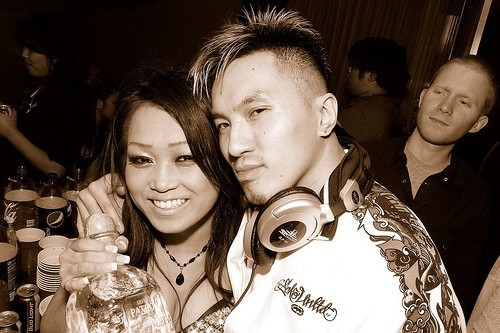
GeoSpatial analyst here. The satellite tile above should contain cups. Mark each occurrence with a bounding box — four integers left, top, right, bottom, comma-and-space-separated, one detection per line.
36, 248, 65, 321
38, 235, 70, 252
16, 227, 46, 272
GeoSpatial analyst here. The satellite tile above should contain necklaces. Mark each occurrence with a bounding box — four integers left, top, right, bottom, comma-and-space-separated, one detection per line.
158, 234, 210, 286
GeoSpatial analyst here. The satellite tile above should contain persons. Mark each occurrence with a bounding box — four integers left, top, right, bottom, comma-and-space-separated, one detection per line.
80, 79, 121, 175
75, 6, 466, 333
335, 34, 413, 146
0, 10, 91, 182
37, 59, 252, 333
365, 51, 500, 321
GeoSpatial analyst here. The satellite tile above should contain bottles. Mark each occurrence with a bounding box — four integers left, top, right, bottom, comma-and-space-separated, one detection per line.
65, 213, 176, 333
35, 172, 77, 239
0, 216, 19, 313
2, 164, 39, 230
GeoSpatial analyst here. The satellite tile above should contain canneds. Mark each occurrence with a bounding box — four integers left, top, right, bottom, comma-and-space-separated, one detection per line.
0, 311, 22, 333
0, 105, 12, 116
14, 284, 41, 333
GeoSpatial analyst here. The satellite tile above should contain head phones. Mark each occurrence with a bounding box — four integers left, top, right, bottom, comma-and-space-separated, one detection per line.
241, 132, 375, 270
